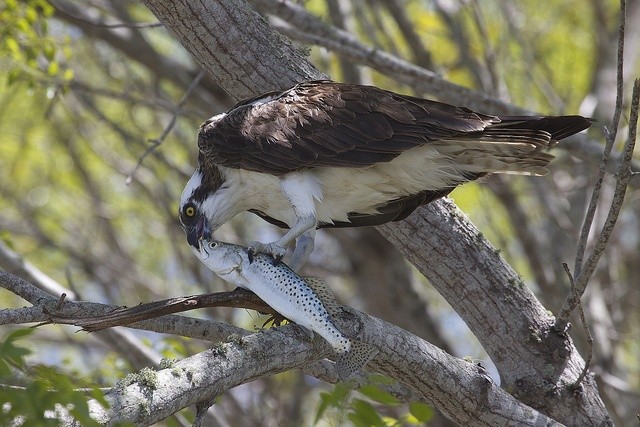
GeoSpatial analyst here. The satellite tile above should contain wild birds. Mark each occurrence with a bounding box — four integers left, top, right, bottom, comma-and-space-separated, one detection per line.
177, 79, 597, 268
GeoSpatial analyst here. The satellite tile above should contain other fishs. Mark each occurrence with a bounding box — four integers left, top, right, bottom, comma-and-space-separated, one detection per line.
188, 238, 380, 383
